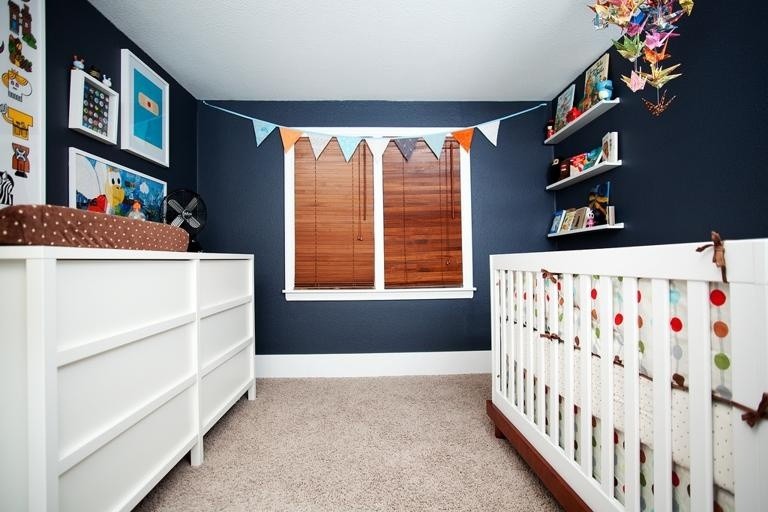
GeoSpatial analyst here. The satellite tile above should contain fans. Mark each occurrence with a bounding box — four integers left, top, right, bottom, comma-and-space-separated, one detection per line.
158, 189, 206, 240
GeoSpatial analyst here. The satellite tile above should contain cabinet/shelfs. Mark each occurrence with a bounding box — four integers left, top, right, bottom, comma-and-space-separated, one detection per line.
1, 247, 254, 511
544, 91, 626, 238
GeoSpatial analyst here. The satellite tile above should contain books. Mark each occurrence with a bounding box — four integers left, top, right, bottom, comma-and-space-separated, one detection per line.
570, 132, 618, 178
583, 53, 610, 98
549, 181, 616, 234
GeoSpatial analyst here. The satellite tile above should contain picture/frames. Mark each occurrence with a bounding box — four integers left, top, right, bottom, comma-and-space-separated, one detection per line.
70, 69, 120, 145
120, 46, 172, 169
67, 147, 168, 221
